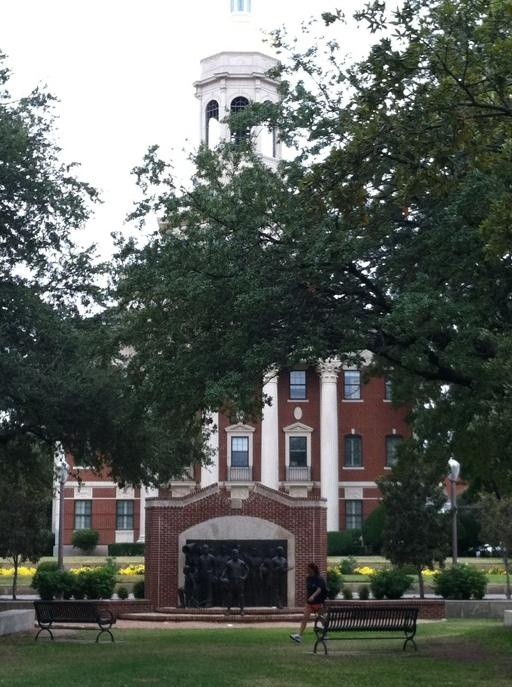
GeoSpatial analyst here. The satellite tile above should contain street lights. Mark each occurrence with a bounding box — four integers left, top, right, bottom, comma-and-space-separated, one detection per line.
447, 458, 463, 562
55, 457, 68, 568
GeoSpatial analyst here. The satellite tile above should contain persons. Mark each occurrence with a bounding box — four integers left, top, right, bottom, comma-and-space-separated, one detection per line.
219, 548, 250, 615
175, 565, 200, 608
269, 545, 294, 609
289, 561, 328, 644
197, 543, 218, 608
182, 541, 275, 606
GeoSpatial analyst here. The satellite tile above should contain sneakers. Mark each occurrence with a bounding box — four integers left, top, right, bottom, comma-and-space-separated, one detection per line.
319, 633, 327, 640
289, 634, 302, 644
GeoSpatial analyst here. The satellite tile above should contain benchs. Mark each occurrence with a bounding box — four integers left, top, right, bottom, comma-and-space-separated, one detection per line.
33, 600, 117, 644
313, 606, 419, 654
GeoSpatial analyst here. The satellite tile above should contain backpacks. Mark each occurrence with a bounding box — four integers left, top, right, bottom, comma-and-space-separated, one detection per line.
320, 576, 328, 601
